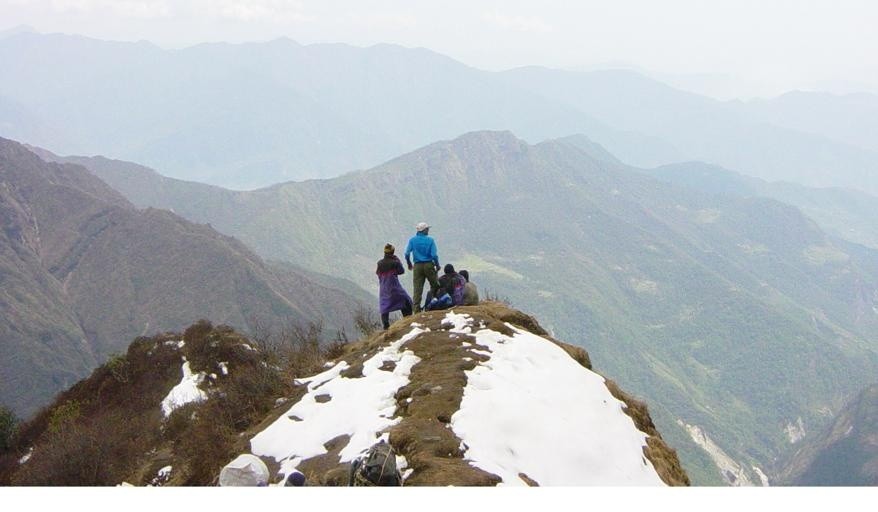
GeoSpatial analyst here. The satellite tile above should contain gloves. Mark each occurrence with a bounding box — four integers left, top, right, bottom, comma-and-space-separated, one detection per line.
408, 264, 413, 271
435, 262, 441, 272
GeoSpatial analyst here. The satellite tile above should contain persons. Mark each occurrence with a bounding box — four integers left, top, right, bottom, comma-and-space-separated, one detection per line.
376, 243, 412, 330
405, 222, 478, 314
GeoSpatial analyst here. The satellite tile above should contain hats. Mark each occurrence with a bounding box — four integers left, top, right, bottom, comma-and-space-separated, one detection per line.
384, 243, 395, 254
444, 264, 454, 274
459, 270, 469, 282
416, 223, 432, 231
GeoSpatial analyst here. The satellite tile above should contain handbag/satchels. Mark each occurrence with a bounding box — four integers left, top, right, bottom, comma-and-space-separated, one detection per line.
422, 288, 454, 311
